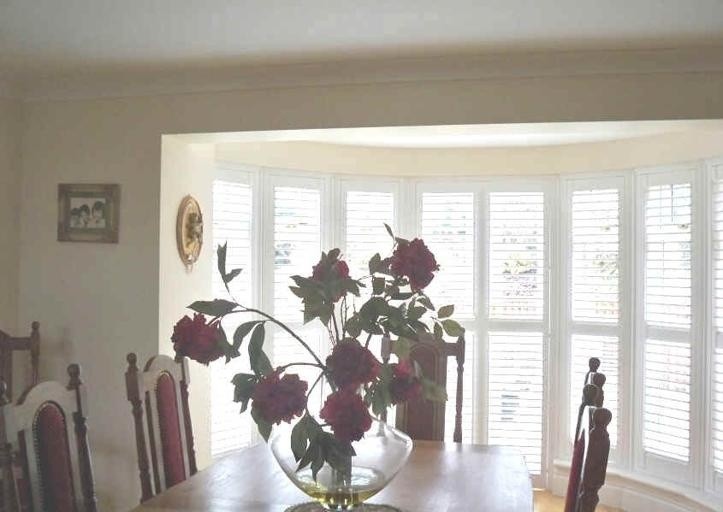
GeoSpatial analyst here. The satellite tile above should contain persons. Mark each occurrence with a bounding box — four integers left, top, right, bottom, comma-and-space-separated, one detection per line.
83, 200, 106, 229
73, 204, 90, 228
70, 207, 80, 227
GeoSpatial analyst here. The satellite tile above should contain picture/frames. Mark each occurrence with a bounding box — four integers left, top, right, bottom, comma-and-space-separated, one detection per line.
57, 182, 121, 245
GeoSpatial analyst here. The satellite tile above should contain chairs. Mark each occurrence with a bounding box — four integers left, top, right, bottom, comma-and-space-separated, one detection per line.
375, 325, 469, 444
563, 355, 613, 512
0, 320, 198, 511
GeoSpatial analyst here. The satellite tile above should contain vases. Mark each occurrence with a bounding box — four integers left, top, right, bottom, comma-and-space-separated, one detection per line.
270, 409, 415, 510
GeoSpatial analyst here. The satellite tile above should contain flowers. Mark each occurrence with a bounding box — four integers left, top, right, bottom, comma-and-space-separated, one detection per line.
170, 220, 466, 489
